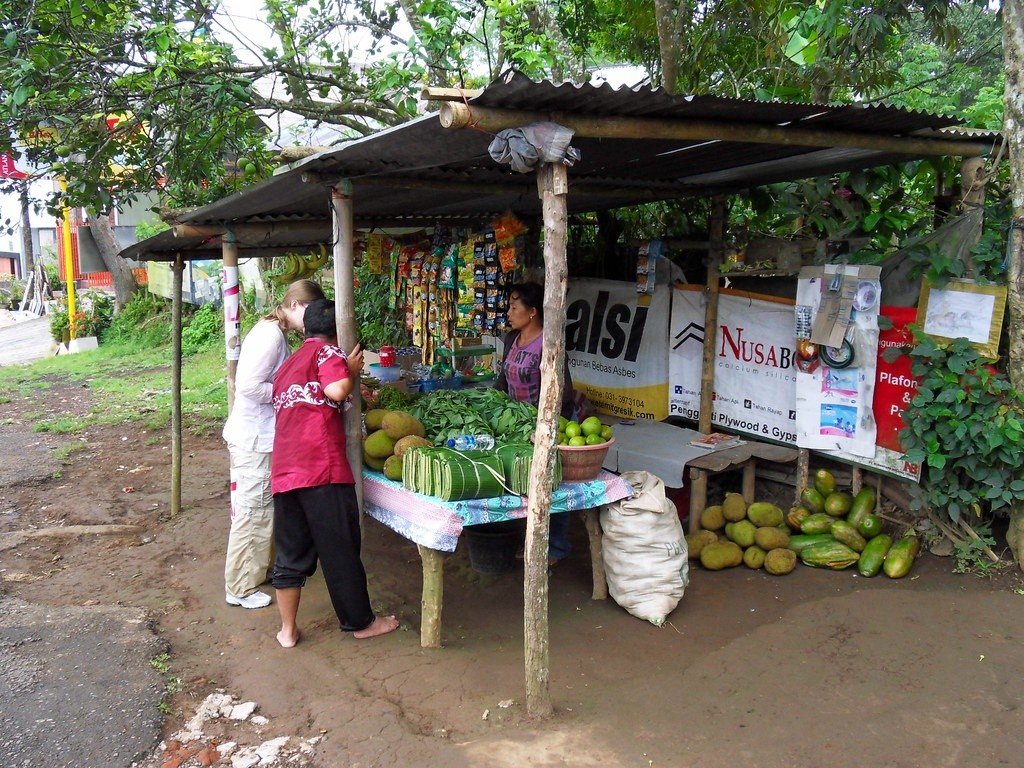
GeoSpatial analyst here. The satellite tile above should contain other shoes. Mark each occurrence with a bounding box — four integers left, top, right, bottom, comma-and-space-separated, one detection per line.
547, 551, 570, 570
513, 547, 524, 561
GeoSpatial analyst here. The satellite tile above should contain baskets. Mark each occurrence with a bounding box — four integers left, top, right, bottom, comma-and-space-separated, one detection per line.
417, 375, 464, 395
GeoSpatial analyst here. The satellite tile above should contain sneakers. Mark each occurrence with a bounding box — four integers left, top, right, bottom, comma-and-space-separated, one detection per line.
225, 591, 273, 610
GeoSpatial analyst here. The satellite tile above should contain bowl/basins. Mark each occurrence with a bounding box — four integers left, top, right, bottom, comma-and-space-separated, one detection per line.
368, 362, 400, 381
407, 383, 420, 394
433, 377, 449, 392
449, 375, 462, 392
419, 379, 435, 394
851, 280, 878, 313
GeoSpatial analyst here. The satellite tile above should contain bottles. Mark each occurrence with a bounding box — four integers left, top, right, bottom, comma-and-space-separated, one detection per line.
447, 434, 495, 451
393, 346, 420, 356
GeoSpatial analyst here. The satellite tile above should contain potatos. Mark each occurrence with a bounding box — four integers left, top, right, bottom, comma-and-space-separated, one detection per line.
364, 409, 432, 480
685, 494, 796, 573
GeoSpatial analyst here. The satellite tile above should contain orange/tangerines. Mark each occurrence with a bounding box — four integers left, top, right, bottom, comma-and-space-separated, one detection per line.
557, 416, 612, 445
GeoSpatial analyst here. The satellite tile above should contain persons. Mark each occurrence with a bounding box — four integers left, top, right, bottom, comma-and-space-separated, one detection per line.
493, 283, 573, 422
222, 280, 399, 648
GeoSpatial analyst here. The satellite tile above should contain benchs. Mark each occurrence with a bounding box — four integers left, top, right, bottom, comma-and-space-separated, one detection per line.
599, 416, 799, 535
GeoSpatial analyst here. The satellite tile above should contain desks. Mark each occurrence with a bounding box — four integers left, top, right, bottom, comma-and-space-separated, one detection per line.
362, 466, 639, 647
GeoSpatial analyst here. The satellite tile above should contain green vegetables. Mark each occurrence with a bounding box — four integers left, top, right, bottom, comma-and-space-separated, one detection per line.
376, 385, 538, 450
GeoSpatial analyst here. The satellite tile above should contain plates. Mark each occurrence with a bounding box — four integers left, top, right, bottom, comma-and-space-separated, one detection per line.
819, 337, 855, 369
